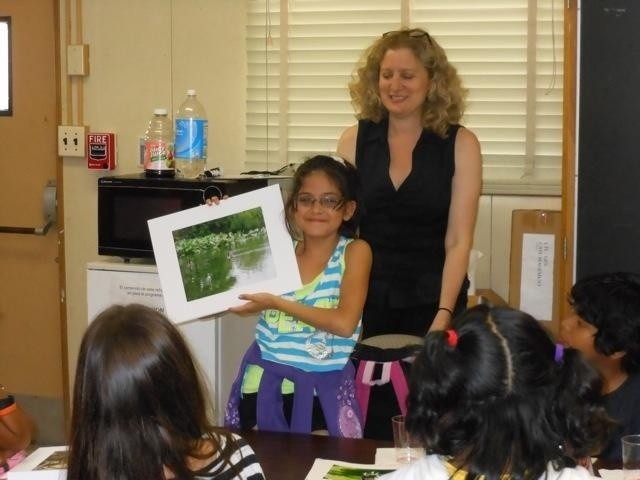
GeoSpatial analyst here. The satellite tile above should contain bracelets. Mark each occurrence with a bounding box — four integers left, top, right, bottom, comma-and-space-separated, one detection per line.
438, 307, 453, 315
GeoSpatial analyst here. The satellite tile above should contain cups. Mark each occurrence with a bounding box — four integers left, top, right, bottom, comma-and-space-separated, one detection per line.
391, 414, 425, 466
619, 433, 640, 480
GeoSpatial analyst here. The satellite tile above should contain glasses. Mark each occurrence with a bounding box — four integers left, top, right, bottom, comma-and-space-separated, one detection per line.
294, 194, 339, 208
383, 30, 432, 45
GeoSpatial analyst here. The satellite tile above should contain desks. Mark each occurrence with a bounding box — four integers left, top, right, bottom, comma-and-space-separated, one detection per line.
214, 425, 640, 480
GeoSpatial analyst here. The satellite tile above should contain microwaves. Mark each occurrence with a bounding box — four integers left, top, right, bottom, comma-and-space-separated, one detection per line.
95, 167, 297, 264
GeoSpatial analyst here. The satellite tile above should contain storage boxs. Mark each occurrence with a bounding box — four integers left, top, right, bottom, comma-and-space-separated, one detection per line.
510, 208, 564, 342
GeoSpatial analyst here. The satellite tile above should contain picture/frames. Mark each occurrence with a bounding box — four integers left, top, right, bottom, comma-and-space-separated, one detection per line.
146, 182, 303, 330
305, 457, 403, 480
6, 446, 72, 480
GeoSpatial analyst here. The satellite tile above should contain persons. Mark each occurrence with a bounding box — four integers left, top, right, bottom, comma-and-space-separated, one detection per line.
374, 302, 624, 480
206, 154, 374, 436
65, 302, 268, 480
335, 27, 485, 337
558, 270, 640, 470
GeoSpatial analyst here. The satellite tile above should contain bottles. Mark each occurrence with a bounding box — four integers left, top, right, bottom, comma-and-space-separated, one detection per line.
143, 107, 174, 177
173, 88, 209, 179
